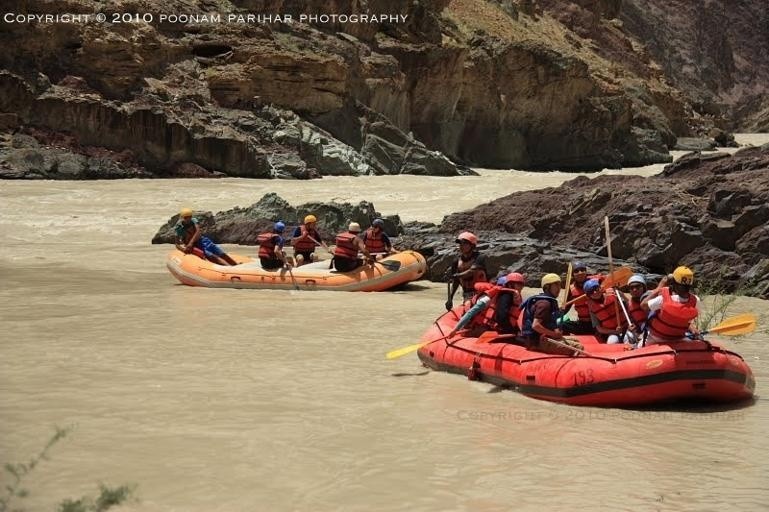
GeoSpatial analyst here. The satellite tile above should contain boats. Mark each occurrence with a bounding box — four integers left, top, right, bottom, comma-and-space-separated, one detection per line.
413, 292, 757, 413
164, 243, 430, 293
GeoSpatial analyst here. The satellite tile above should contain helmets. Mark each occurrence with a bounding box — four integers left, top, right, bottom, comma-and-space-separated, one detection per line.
672, 265, 694, 286
372, 219, 384, 225
274, 221, 286, 231
457, 232, 477, 245
304, 215, 316, 225
179, 208, 193, 218
541, 272, 562, 288
583, 278, 600, 294
572, 261, 586, 272
506, 272, 525, 286
348, 222, 361, 232
627, 275, 648, 290
497, 276, 508, 285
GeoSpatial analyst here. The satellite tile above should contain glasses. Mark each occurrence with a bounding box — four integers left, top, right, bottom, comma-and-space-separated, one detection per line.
574, 268, 584, 273
629, 283, 642, 290
588, 287, 599, 294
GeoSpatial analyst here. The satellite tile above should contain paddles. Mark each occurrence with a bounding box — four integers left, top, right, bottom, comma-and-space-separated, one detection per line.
565, 267, 634, 308
384, 327, 473, 361
356, 258, 401, 272
685, 312, 757, 337
560, 263, 573, 331
476, 331, 518, 345
603, 215, 621, 328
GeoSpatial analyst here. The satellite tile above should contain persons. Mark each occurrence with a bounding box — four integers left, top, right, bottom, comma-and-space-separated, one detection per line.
442, 228, 703, 356
258, 221, 295, 269
289, 214, 335, 267
331, 221, 374, 272
173, 208, 244, 266
360, 217, 400, 265
